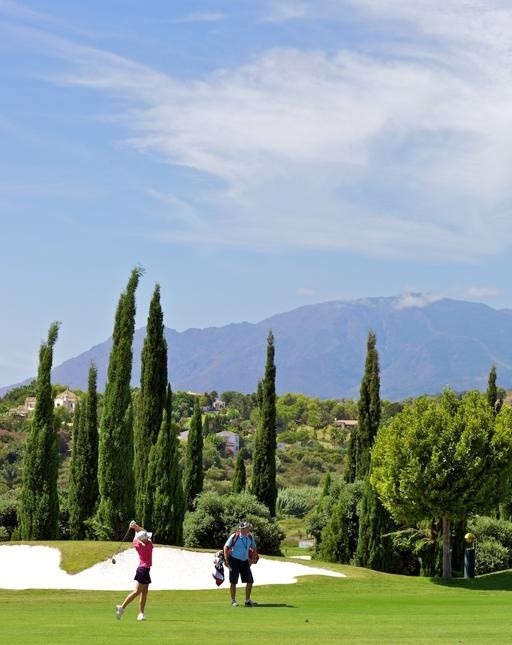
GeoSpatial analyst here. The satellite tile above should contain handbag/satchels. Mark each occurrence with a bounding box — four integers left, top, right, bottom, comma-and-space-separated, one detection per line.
248, 548, 259, 564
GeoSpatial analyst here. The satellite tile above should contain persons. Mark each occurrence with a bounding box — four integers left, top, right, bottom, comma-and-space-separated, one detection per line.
116, 522, 153, 621
223, 522, 257, 607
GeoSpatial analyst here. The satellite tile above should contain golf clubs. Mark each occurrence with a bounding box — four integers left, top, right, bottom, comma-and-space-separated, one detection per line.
112, 528, 131, 564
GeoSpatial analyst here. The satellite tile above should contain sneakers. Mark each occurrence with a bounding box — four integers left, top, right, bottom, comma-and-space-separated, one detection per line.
137, 613, 145, 620
232, 601, 257, 606
116, 605, 123, 618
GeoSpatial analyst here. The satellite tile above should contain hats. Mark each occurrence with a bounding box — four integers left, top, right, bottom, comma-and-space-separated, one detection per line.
239, 522, 249, 528
137, 531, 152, 540
